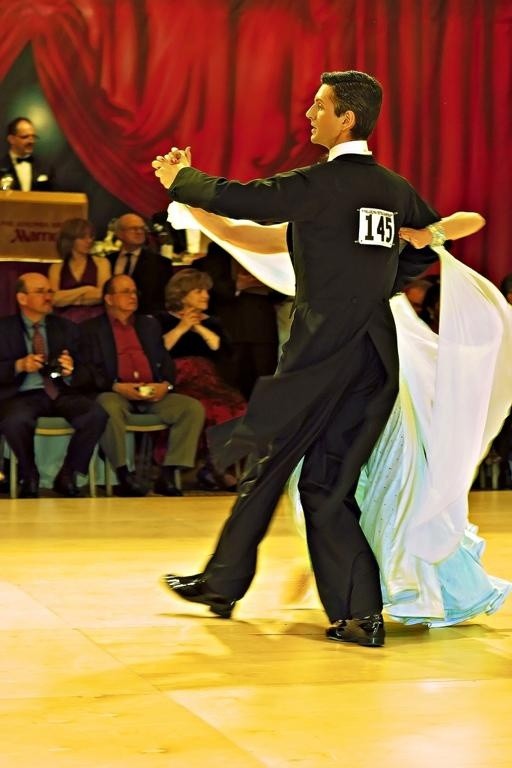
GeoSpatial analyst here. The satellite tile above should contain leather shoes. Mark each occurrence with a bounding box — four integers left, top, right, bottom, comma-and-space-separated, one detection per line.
326, 613, 384, 646
119, 472, 148, 497
55, 471, 86, 497
197, 466, 223, 491
154, 479, 183, 496
165, 573, 235, 619
19, 466, 39, 499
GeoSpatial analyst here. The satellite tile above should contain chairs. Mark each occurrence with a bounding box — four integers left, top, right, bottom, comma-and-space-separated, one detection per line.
9, 416, 98, 497
104, 412, 185, 496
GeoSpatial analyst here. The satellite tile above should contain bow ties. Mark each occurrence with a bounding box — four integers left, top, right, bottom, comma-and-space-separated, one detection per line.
17, 155, 34, 164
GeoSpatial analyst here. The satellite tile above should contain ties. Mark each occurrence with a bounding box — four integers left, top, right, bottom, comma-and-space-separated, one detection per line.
124, 253, 132, 275
32, 323, 60, 401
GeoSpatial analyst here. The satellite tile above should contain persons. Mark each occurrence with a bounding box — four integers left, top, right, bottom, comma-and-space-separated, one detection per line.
406, 274, 512, 489
83, 274, 205, 497
48, 218, 112, 321
108, 215, 175, 315
1, 273, 109, 498
0, 117, 68, 191
151, 70, 451, 647
146, 243, 295, 492
153, 144, 512, 630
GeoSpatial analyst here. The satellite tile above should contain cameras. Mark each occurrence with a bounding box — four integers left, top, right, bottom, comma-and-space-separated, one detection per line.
138, 386, 155, 398
37, 359, 68, 382
